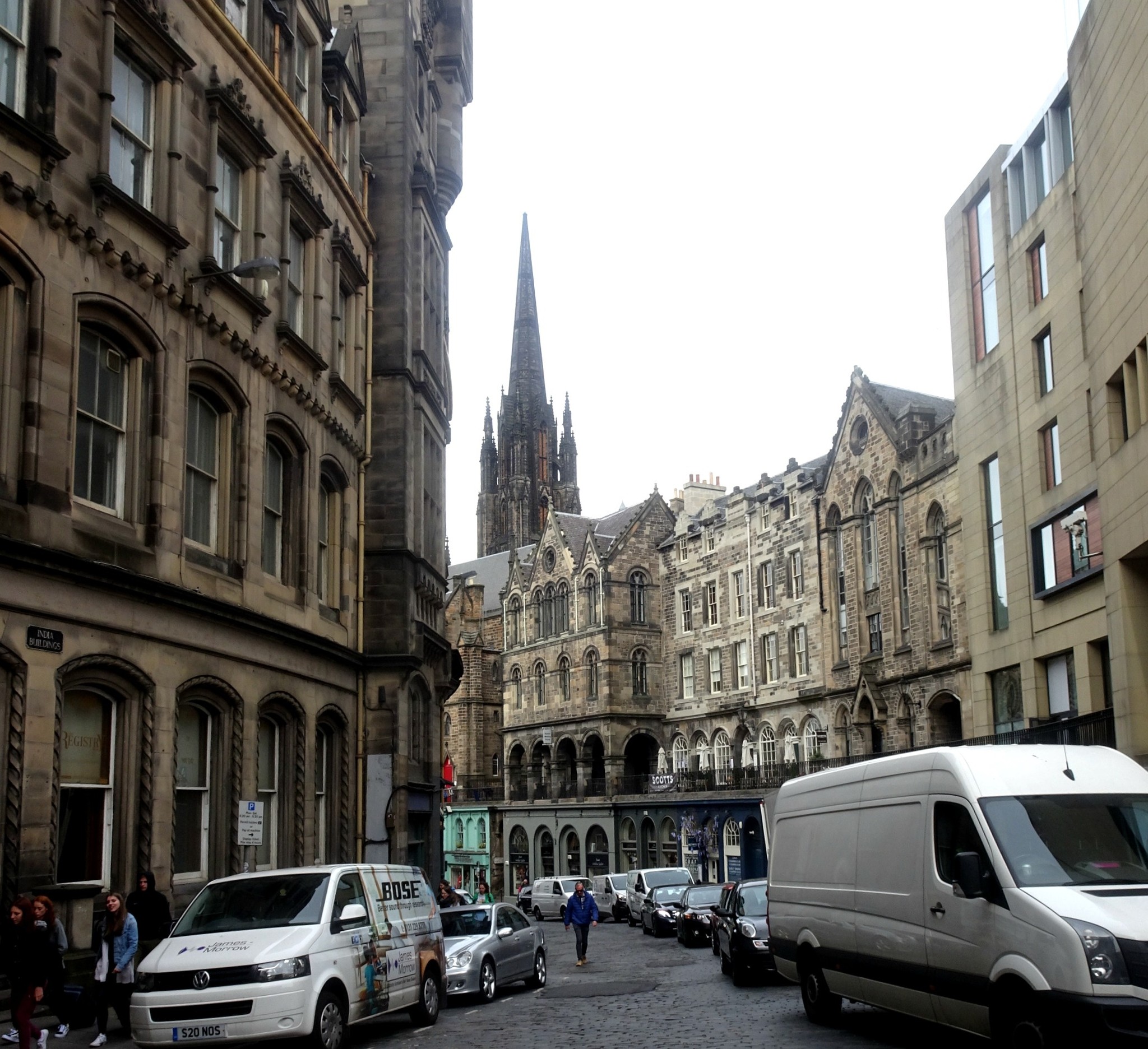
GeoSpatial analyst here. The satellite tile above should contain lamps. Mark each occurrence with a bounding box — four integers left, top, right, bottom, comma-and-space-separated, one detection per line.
897, 673, 921, 708
183, 257, 280, 286
738, 705, 755, 730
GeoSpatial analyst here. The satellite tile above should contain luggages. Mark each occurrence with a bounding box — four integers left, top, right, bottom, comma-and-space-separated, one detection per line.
49, 985, 96, 1030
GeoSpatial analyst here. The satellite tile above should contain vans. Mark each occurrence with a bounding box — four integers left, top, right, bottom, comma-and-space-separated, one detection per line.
129, 863, 448, 1049
760, 744, 1148, 1049
593, 874, 629, 923
626, 867, 696, 928
531, 876, 594, 921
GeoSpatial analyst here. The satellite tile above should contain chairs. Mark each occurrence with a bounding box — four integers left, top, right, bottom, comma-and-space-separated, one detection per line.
745, 889, 758, 906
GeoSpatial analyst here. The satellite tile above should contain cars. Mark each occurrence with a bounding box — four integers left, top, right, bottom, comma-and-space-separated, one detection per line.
718, 878, 788, 986
515, 886, 533, 915
709, 883, 735, 956
640, 883, 695, 939
439, 903, 548, 1002
673, 882, 728, 947
454, 889, 473, 904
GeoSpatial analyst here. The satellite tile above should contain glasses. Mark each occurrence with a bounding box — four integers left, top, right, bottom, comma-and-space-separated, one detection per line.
576, 887, 584, 893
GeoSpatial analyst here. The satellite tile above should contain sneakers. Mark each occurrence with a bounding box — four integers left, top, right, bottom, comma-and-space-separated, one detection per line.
90, 1033, 107, 1046
2, 1029, 19, 1042
36, 1030, 49, 1049
54, 1023, 69, 1038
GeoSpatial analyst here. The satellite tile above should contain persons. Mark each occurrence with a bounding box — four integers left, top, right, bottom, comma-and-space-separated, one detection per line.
564, 882, 598, 966
89, 892, 138, 1047
126, 871, 172, 972
386, 923, 405, 948
472, 882, 494, 903
1, 895, 70, 1042
361, 951, 377, 1010
457, 875, 462, 889
437, 880, 450, 906
517, 879, 527, 898
6, 898, 50, 1049
441, 886, 461, 908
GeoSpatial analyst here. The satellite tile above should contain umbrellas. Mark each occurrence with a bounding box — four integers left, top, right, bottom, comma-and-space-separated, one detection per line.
657, 746, 668, 774
784, 735, 797, 770
740, 739, 753, 780
698, 745, 710, 775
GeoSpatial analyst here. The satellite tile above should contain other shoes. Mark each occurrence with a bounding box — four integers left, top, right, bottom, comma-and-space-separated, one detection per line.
582, 958, 586, 964
576, 960, 582, 966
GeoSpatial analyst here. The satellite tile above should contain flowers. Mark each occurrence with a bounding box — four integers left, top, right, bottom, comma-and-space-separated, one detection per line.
671, 815, 719, 869
